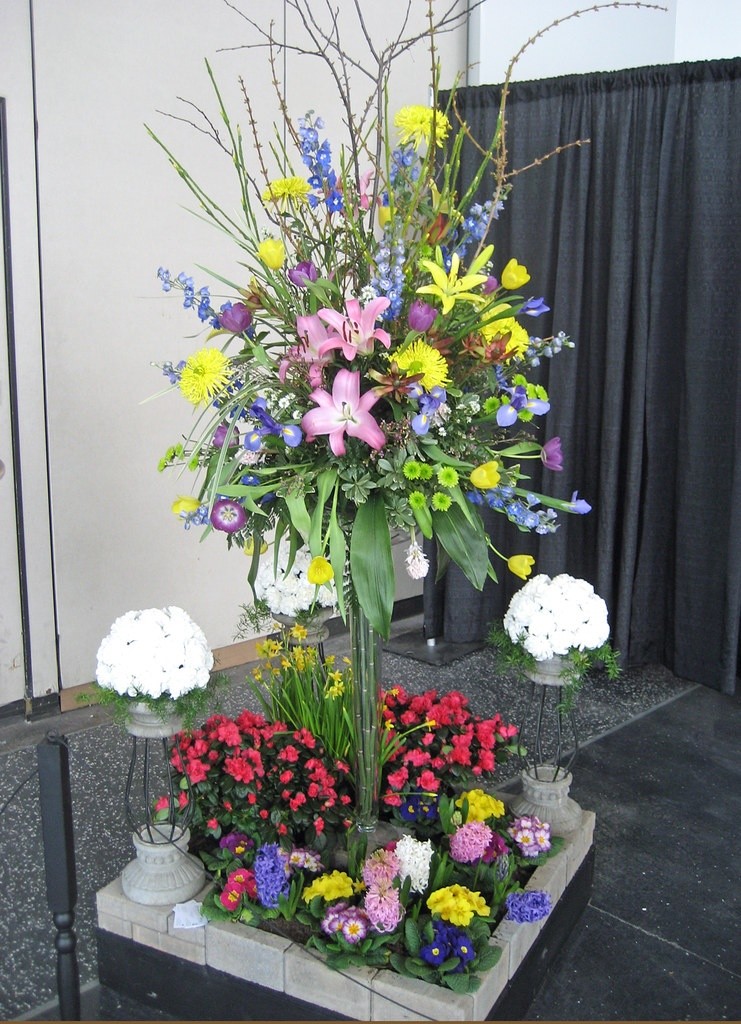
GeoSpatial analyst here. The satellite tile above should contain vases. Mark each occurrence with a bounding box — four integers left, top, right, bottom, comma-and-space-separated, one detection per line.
342, 605, 401, 851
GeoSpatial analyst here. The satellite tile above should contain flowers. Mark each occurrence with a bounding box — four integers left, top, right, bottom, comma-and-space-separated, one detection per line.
238, 534, 355, 648
483, 570, 624, 715
167, 624, 573, 993
132, 0, 670, 645
72, 606, 234, 738
